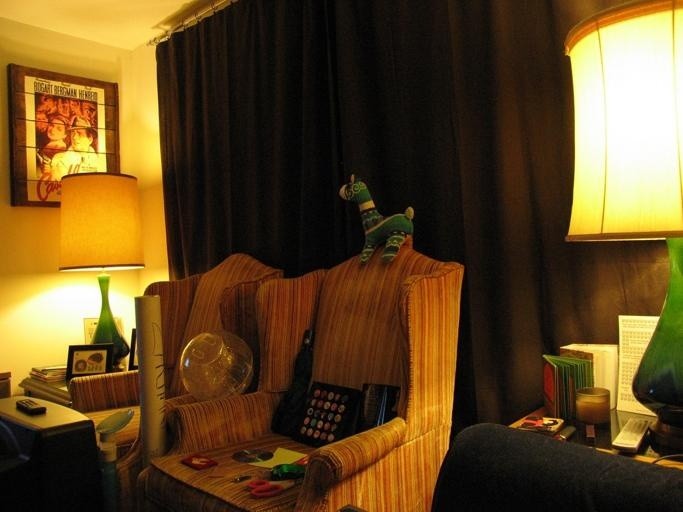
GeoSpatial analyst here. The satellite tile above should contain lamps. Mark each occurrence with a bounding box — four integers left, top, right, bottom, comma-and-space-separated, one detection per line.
58, 169, 146, 374
557, 2, 681, 458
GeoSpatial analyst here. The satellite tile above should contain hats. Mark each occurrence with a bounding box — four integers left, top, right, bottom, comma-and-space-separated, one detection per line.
66, 116, 94, 130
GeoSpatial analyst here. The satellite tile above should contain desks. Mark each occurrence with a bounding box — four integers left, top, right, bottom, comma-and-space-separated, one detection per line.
18, 364, 149, 407
499, 390, 682, 473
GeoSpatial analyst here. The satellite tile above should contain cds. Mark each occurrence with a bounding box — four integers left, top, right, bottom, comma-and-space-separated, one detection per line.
232, 449, 273, 463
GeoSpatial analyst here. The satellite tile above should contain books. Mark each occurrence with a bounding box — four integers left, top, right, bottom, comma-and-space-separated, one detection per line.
615, 315, 662, 419
28, 370, 65, 381
30, 375, 64, 383
557, 340, 620, 412
541, 353, 592, 422
31, 364, 66, 374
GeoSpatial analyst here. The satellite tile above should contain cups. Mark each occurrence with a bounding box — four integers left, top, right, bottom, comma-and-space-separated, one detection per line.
574, 388, 610, 426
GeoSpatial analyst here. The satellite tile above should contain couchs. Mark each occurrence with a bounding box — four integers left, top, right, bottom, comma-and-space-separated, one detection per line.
62, 251, 288, 511
139, 243, 473, 511
429, 418, 681, 511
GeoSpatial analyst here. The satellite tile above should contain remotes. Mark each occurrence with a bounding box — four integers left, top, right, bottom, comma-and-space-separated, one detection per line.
16, 399, 46, 414
612, 417, 652, 453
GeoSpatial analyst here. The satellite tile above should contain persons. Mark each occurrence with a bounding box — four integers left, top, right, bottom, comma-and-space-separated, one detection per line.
49, 117, 99, 175
35, 111, 48, 147
39, 114, 71, 160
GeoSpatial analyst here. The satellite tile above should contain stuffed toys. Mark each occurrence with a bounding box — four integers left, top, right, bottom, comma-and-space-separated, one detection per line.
337, 173, 416, 267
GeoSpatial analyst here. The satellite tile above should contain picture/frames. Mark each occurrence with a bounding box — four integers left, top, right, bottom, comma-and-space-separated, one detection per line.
5, 62, 122, 211
